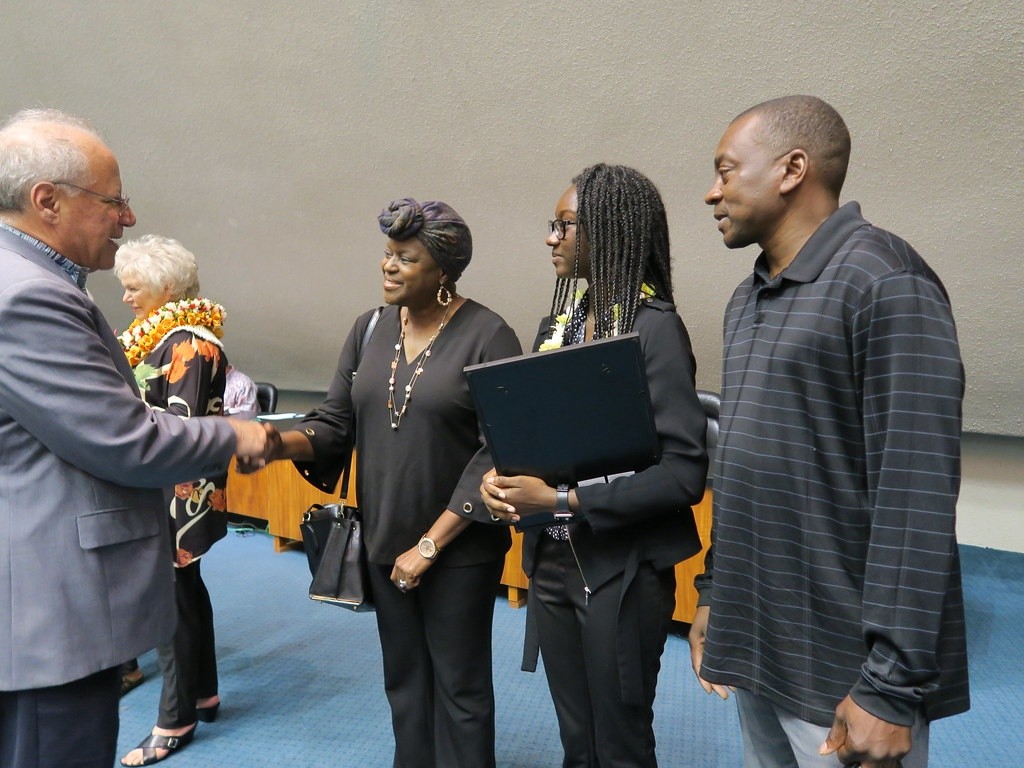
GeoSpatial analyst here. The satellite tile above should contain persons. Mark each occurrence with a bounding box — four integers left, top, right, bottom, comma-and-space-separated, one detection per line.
685, 94, 975, 768
473, 159, 709, 767
1, 102, 274, 768
99, 232, 229, 768
112, 658, 148, 700
231, 194, 525, 768
219, 350, 260, 420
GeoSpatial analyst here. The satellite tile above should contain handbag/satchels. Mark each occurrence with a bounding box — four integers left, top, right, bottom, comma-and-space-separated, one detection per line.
300, 499, 376, 613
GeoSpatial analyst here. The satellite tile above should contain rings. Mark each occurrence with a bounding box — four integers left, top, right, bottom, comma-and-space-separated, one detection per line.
398, 579, 407, 589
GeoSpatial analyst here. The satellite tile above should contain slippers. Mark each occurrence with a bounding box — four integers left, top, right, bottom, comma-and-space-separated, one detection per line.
119, 671, 144, 698
196, 701, 220, 723
120, 717, 199, 767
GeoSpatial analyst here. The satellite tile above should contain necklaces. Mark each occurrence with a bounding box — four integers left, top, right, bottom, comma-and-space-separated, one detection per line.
587, 311, 597, 324
385, 296, 452, 430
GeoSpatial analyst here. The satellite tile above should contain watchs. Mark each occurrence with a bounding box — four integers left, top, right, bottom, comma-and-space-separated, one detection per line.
418, 533, 441, 560
552, 483, 575, 522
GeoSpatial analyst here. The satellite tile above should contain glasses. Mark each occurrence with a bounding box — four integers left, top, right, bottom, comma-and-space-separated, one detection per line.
547, 219, 576, 240
53, 181, 130, 217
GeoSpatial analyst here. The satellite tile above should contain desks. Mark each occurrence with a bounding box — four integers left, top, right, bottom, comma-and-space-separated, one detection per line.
224, 410, 714, 630
